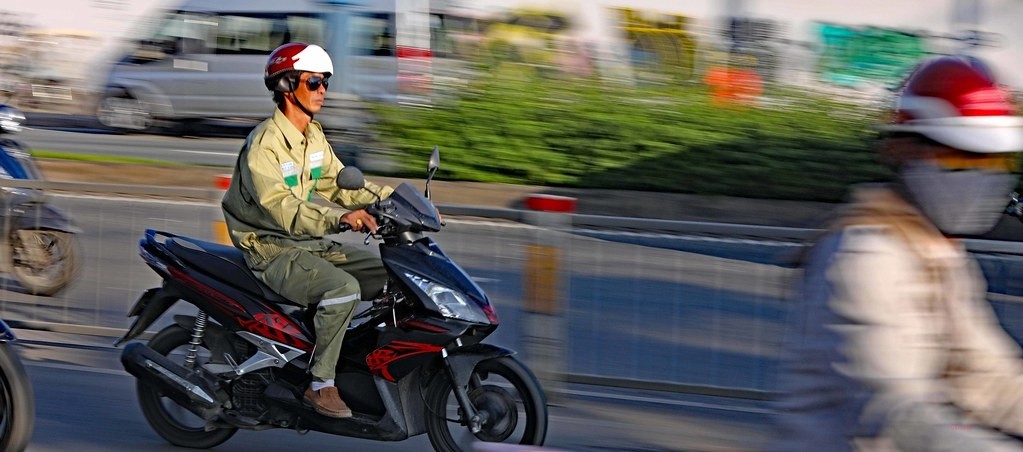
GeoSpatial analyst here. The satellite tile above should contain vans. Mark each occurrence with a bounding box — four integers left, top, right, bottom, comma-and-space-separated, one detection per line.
94, 1, 520, 133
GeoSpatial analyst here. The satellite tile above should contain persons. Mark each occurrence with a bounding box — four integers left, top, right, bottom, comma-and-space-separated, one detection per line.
222, 44, 442, 421
778, 58, 1022, 452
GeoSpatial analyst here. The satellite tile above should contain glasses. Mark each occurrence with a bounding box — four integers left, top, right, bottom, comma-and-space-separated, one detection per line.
301, 76, 329, 92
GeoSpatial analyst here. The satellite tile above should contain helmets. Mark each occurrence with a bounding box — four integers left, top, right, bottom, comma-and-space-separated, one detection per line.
265, 43, 334, 77
874, 59, 1023, 151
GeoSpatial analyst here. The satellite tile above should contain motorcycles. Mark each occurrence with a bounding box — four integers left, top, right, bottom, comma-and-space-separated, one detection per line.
113, 146, 551, 452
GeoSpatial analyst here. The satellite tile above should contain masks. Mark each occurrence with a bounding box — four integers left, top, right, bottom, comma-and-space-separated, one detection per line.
900, 161, 1021, 234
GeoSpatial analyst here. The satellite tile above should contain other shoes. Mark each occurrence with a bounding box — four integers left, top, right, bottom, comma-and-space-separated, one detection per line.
302, 386, 352, 417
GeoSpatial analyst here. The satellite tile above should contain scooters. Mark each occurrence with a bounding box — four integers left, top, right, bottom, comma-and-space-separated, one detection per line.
0, 105, 86, 297
